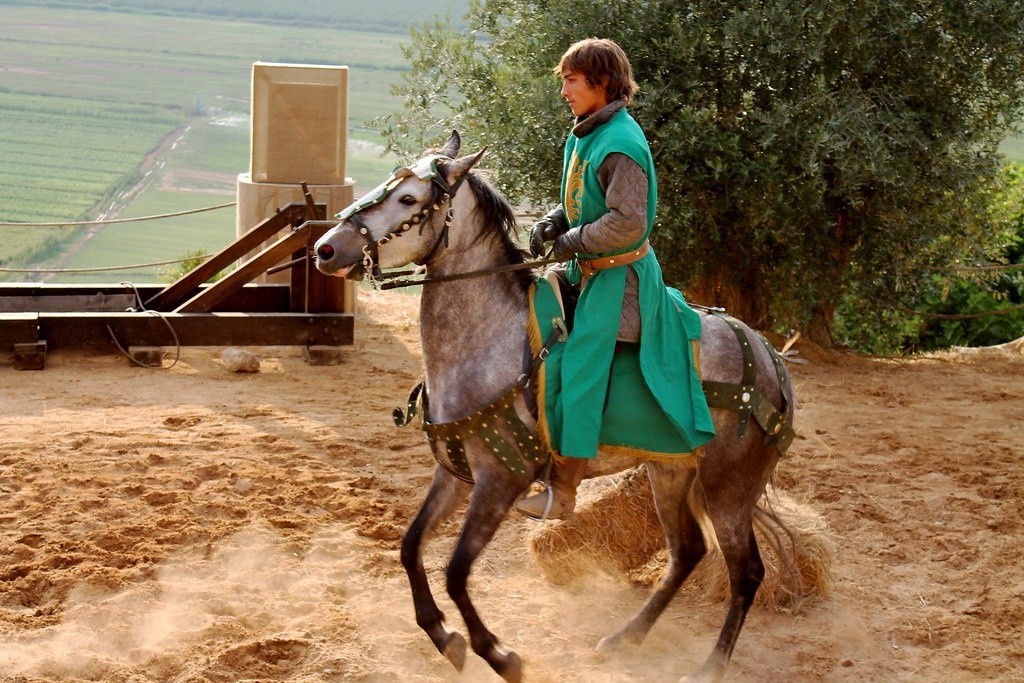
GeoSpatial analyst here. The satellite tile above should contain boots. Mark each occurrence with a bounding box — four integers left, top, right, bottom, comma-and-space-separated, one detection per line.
516, 456, 589, 521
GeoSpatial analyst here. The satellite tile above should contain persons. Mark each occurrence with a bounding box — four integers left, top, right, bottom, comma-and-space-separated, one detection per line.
516, 39, 717, 520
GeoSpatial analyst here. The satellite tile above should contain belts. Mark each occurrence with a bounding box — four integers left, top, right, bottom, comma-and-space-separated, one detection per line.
576, 238, 651, 292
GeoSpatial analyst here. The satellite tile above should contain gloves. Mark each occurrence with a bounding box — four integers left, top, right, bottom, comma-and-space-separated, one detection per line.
529, 209, 570, 258
554, 222, 594, 263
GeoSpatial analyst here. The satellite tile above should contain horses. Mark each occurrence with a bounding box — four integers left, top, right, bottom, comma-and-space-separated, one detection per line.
312, 129, 802, 683
